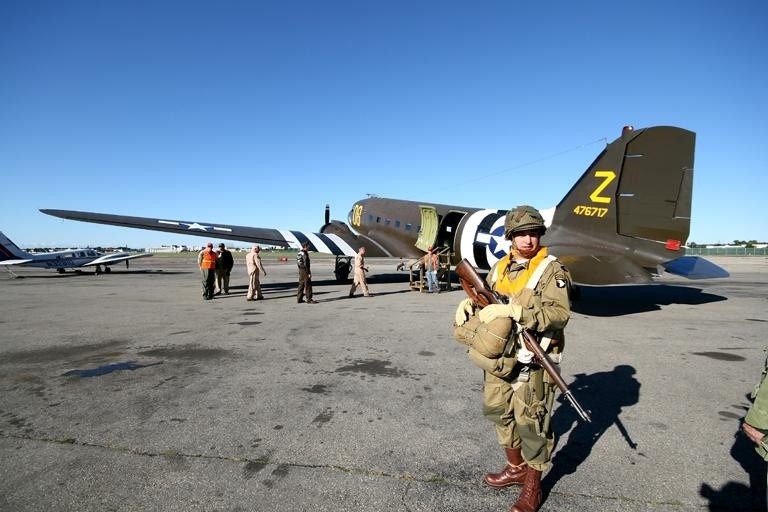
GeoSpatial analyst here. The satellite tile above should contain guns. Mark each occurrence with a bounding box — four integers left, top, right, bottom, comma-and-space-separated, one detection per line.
454, 259, 592, 424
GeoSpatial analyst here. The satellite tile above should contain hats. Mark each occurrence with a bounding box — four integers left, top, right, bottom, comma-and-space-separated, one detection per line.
219, 243, 224, 247
208, 243, 214, 248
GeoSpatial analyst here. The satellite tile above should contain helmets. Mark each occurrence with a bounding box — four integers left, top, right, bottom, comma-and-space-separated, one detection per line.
504, 205, 546, 240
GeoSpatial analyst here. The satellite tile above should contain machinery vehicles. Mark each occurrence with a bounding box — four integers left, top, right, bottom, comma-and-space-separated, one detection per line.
145, 244, 199, 253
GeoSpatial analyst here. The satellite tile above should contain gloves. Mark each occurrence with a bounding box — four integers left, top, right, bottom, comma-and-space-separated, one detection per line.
455, 297, 474, 327
478, 303, 523, 324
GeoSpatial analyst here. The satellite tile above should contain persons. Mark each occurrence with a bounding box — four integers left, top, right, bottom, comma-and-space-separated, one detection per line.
215, 243, 234, 296
197, 242, 218, 299
245, 246, 266, 301
296, 240, 318, 304
349, 247, 374, 298
456, 205, 572, 512
737, 346, 768, 512
424, 246, 441, 294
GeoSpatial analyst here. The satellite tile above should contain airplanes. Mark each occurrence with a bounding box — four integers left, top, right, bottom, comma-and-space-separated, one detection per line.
38, 125, 730, 288
0, 230, 153, 276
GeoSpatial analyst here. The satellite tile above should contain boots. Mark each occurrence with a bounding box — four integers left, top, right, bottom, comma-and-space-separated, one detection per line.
509, 464, 544, 512
485, 447, 527, 487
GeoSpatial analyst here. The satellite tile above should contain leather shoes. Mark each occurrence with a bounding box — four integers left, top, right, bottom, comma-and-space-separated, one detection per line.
306, 299, 317, 303
257, 296, 263, 299
349, 295, 357, 297
248, 298, 256, 301
298, 299, 305, 303
